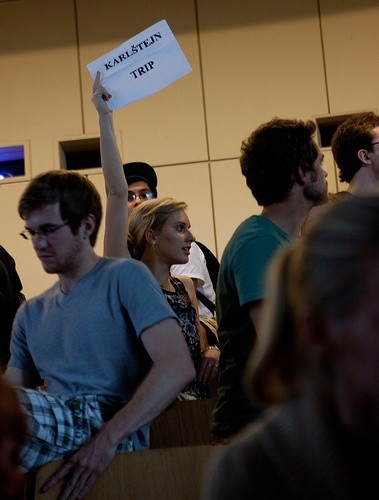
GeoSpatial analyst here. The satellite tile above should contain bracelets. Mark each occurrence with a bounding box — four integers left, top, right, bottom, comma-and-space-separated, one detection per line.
205, 345, 222, 353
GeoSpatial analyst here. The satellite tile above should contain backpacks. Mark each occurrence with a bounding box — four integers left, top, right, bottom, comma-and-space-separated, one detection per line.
194, 239, 220, 317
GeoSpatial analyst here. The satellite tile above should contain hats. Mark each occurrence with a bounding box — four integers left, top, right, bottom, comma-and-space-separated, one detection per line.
123, 162, 157, 189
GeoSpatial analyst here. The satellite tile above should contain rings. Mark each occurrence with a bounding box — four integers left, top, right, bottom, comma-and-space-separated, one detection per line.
215, 364, 219, 368
208, 364, 213, 367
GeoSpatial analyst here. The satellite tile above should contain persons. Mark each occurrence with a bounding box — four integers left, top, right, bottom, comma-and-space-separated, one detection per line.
91, 71, 222, 448
0, 111, 379, 500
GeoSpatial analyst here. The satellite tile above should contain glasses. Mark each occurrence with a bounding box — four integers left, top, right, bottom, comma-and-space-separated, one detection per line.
25, 222, 73, 239
128, 190, 154, 202
